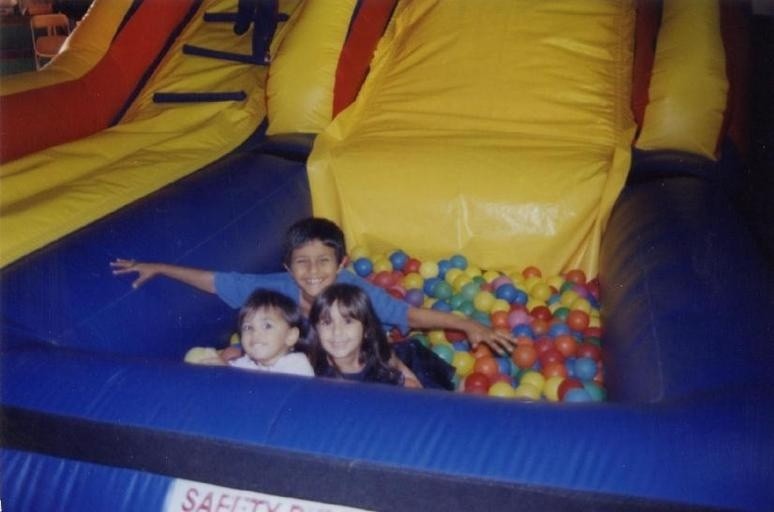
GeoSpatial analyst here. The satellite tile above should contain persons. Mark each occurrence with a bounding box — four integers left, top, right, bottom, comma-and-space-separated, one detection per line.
107, 215, 519, 392
182, 287, 316, 377
231, 0, 279, 64
217, 281, 423, 388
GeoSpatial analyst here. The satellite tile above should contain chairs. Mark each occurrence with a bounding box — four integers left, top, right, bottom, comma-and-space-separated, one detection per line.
30, 13, 71, 71
